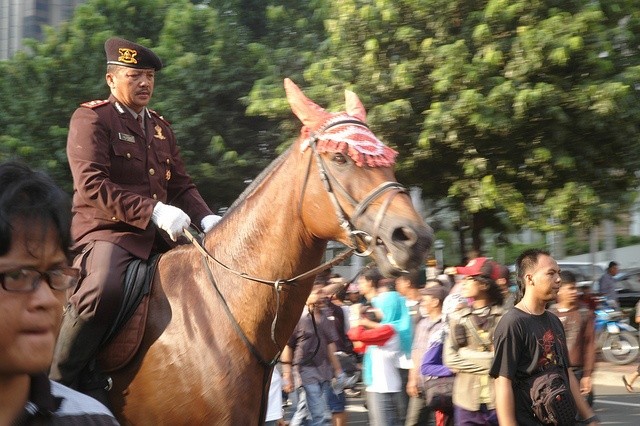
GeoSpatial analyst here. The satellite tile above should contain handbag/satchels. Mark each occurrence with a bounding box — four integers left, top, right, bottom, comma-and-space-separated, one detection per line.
422, 374, 457, 416
530, 313, 578, 425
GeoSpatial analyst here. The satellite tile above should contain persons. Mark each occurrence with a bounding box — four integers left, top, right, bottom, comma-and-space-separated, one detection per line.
49, 37, 222, 407
266, 266, 461, 424
495, 266, 518, 309
545, 271, 595, 425
443, 257, 510, 425
576, 285, 595, 311
622, 299, 640, 392
490, 250, 600, 425
1, 163, 121, 425
600, 261, 620, 308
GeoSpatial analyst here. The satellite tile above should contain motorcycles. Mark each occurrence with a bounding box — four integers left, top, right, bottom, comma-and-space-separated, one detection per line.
590, 296, 639, 365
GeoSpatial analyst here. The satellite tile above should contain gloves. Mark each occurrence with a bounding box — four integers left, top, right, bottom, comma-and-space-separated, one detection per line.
152, 201, 191, 242
200, 214, 223, 233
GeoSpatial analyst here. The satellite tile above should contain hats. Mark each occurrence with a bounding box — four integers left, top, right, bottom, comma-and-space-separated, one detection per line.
457, 257, 501, 281
419, 285, 450, 301
104, 37, 162, 70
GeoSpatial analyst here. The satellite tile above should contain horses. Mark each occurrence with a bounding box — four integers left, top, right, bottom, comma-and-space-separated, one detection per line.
87, 77, 437, 425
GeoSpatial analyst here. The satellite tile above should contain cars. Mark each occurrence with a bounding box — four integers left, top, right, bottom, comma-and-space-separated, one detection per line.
509, 262, 607, 307
584, 267, 640, 302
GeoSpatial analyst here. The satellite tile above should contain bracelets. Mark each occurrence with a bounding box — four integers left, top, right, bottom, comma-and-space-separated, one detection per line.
585, 415, 598, 424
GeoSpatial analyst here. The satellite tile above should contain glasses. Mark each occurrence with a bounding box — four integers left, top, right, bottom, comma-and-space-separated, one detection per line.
0, 267, 81, 292
